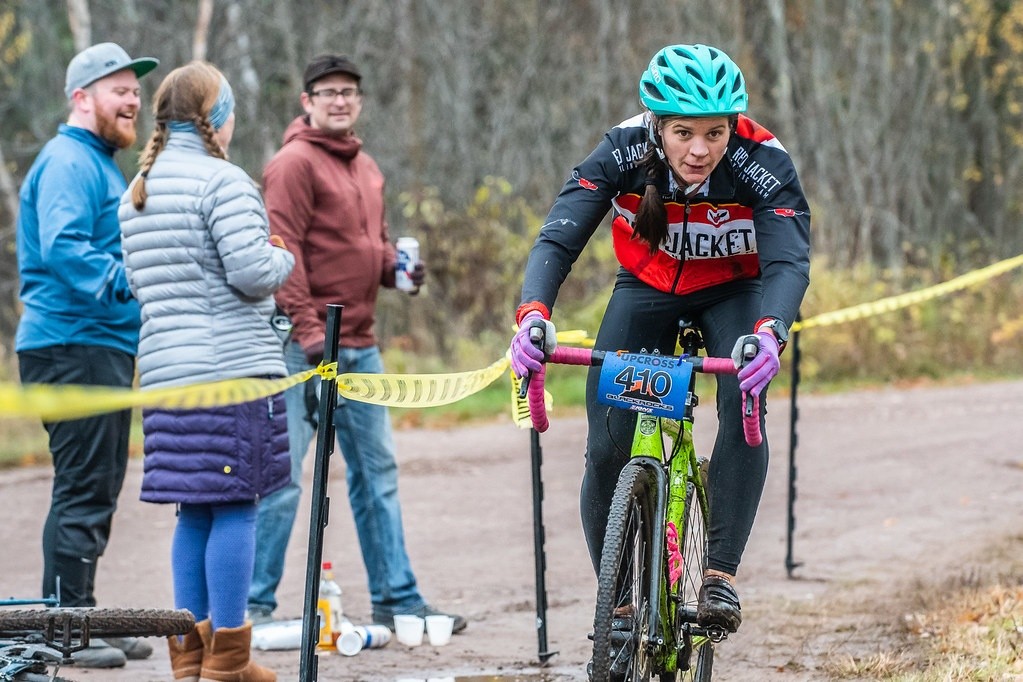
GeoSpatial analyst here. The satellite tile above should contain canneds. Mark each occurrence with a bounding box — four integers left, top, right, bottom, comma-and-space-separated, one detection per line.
395, 237, 419, 291
352, 625, 392, 648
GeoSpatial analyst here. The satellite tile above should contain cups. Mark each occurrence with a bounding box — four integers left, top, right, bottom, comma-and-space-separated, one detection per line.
392, 613, 453, 646
336, 632, 363, 656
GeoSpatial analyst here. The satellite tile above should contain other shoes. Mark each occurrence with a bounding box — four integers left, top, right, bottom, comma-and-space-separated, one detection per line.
102, 637, 152, 660
70, 638, 126, 668
372, 604, 466, 634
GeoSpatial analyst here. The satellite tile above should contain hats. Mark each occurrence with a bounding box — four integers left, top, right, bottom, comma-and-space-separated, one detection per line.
304, 54, 362, 87
64, 43, 160, 100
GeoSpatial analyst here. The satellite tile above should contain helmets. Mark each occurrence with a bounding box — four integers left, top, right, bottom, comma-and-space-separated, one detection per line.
640, 44, 748, 117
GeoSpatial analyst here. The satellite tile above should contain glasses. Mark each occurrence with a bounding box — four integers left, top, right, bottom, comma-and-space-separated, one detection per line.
311, 88, 362, 98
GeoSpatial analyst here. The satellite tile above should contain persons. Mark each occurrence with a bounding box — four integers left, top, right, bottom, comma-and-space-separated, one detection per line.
509, 41, 811, 680
245, 52, 469, 634
16, 40, 160, 667
118, 60, 296, 682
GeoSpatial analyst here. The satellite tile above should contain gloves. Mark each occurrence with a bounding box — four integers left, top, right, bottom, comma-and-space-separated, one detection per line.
732, 332, 780, 397
507, 312, 556, 379
306, 343, 325, 365
408, 263, 423, 295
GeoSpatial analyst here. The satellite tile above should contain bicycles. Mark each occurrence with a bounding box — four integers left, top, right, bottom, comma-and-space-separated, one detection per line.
516, 317, 760, 681
0, 595, 195, 682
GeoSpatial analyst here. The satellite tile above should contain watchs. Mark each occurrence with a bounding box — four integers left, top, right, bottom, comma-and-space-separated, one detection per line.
758, 320, 790, 348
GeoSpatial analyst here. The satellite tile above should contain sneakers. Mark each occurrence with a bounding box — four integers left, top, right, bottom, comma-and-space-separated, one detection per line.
696, 575, 742, 633
586, 618, 637, 682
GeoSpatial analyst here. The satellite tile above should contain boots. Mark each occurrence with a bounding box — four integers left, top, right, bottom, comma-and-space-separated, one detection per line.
195, 620, 277, 682
167, 632, 202, 682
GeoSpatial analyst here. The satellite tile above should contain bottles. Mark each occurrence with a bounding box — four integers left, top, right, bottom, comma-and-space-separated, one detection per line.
315, 562, 342, 651
395, 237, 419, 291
355, 625, 392, 647
